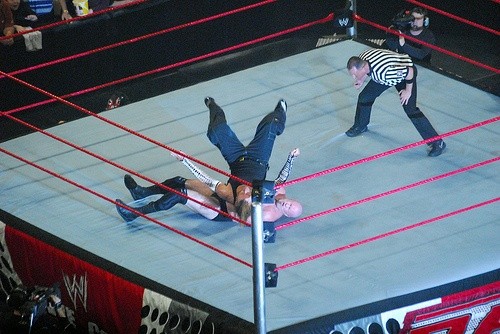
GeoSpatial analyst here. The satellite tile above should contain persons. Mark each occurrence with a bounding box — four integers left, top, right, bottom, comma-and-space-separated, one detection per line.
387, 5, 436, 66
0, 0, 115, 44
0, 286, 88, 334
169, 96, 301, 220
343, 47, 446, 158
114, 173, 303, 225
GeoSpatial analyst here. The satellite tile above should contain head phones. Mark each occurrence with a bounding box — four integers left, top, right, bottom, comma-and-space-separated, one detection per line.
423, 15, 429, 27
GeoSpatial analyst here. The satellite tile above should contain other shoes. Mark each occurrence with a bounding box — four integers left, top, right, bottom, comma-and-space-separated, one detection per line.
204, 96, 215, 109
274, 99, 287, 115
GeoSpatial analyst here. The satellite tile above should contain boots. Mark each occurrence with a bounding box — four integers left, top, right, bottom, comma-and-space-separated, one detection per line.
115, 199, 157, 222
124, 174, 161, 200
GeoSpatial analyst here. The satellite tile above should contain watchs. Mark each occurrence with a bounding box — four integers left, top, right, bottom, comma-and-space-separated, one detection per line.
62, 9, 70, 15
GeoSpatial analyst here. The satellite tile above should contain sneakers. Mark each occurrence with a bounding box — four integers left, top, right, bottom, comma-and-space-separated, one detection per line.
344, 124, 368, 137
429, 140, 447, 157
